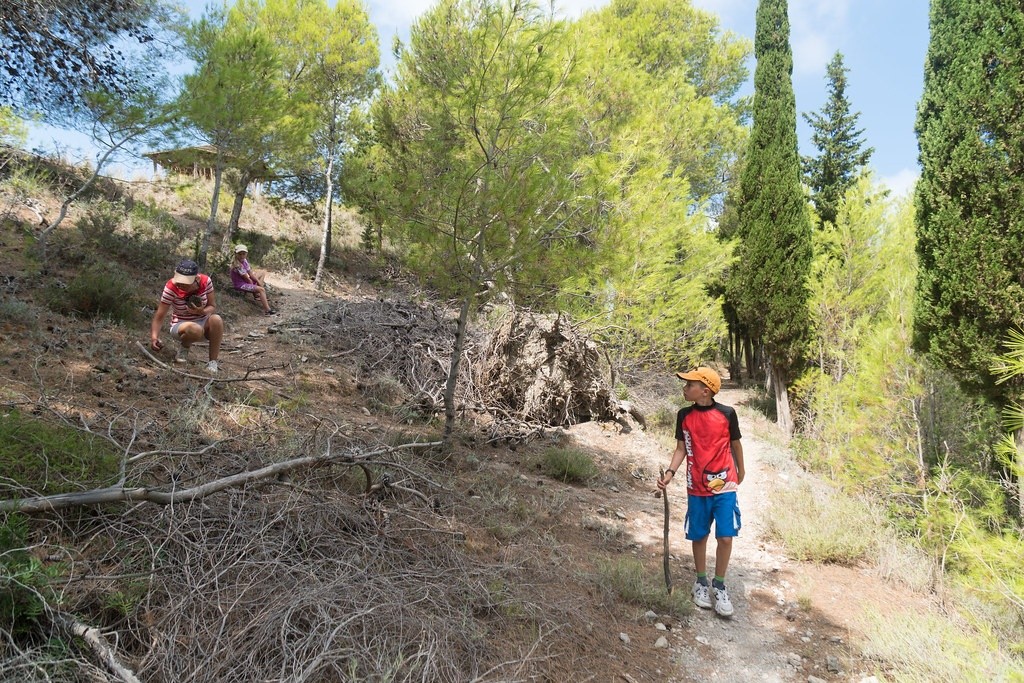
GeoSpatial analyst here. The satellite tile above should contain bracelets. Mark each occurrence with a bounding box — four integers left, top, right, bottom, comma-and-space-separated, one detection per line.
665, 469, 675, 476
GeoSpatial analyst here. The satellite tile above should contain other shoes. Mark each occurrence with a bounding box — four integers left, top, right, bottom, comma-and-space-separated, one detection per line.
265, 310, 277, 317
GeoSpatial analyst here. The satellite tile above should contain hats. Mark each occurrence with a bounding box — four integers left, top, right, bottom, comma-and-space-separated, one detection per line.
677, 367, 721, 394
235, 245, 248, 253
173, 260, 198, 284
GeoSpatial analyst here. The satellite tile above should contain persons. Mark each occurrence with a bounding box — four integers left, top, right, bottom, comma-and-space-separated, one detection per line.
230, 244, 277, 317
151, 260, 224, 371
657, 366, 746, 616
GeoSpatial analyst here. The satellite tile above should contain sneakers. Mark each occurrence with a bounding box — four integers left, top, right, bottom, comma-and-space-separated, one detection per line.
691, 581, 712, 609
175, 344, 189, 362
712, 585, 734, 616
207, 360, 218, 372
